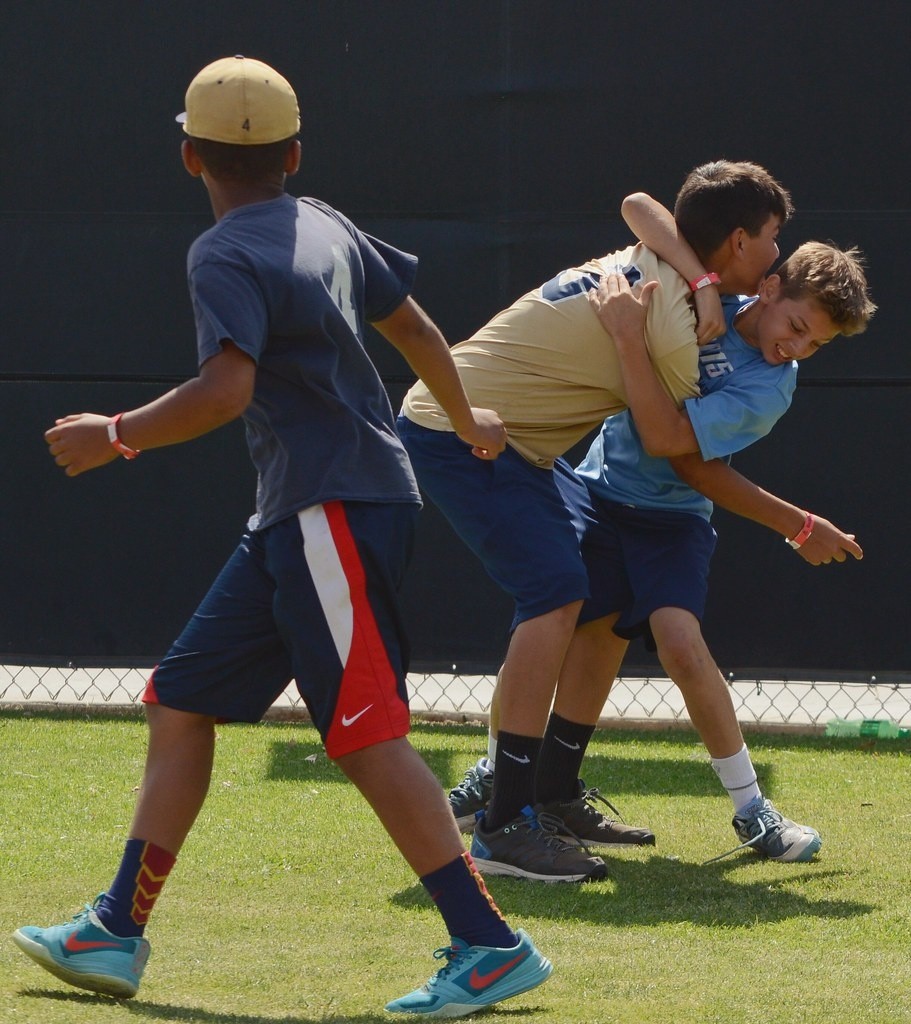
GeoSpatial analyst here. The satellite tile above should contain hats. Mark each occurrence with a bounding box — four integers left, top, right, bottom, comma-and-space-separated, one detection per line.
175, 55, 301, 145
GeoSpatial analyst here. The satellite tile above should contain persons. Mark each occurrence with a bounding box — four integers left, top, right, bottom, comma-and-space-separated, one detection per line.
394, 160, 864, 883
7, 53, 555, 1022
446, 190, 879, 860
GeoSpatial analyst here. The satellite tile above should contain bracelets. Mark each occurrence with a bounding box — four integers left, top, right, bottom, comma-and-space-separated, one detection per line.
784, 510, 816, 550
107, 412, 139, 459
690, 272, 721, 291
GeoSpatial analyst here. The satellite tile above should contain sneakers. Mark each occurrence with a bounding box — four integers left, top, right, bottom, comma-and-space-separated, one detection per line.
469, 803, 609, 885
447, 757, 494, 833
702, 799, 822, 865
12, 892, 150, 998
543, 787, 655, 851
382, 928, 554, 1019
